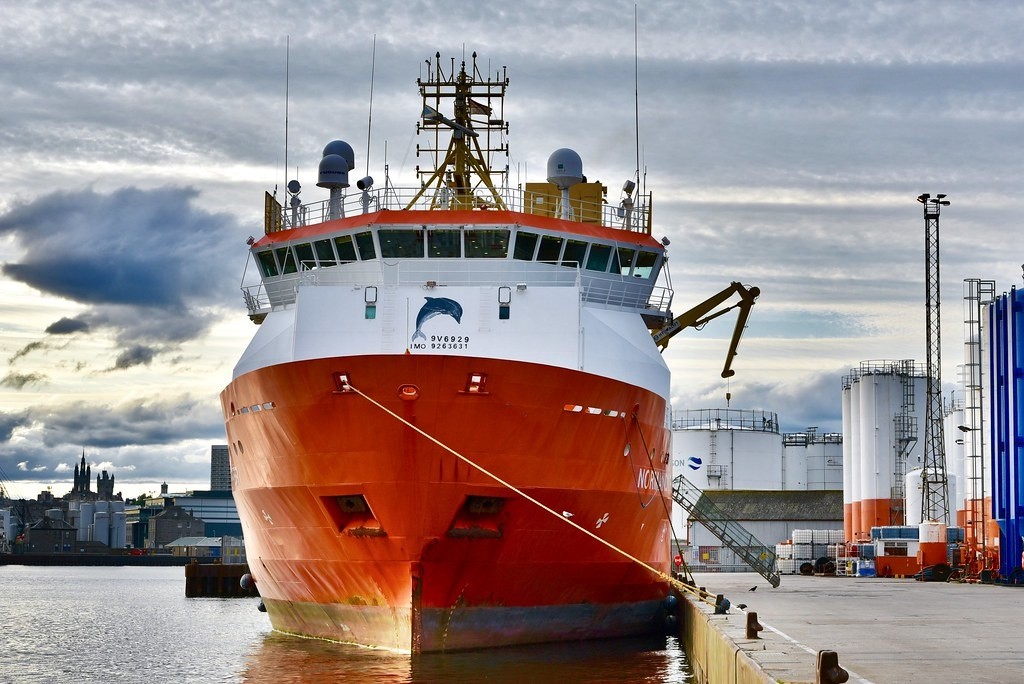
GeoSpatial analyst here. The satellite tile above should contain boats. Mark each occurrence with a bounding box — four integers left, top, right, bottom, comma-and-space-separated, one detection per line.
218, 2, 686, 656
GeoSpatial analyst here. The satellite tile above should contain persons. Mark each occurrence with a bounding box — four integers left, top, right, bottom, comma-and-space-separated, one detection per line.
15, 533, 25, 545
